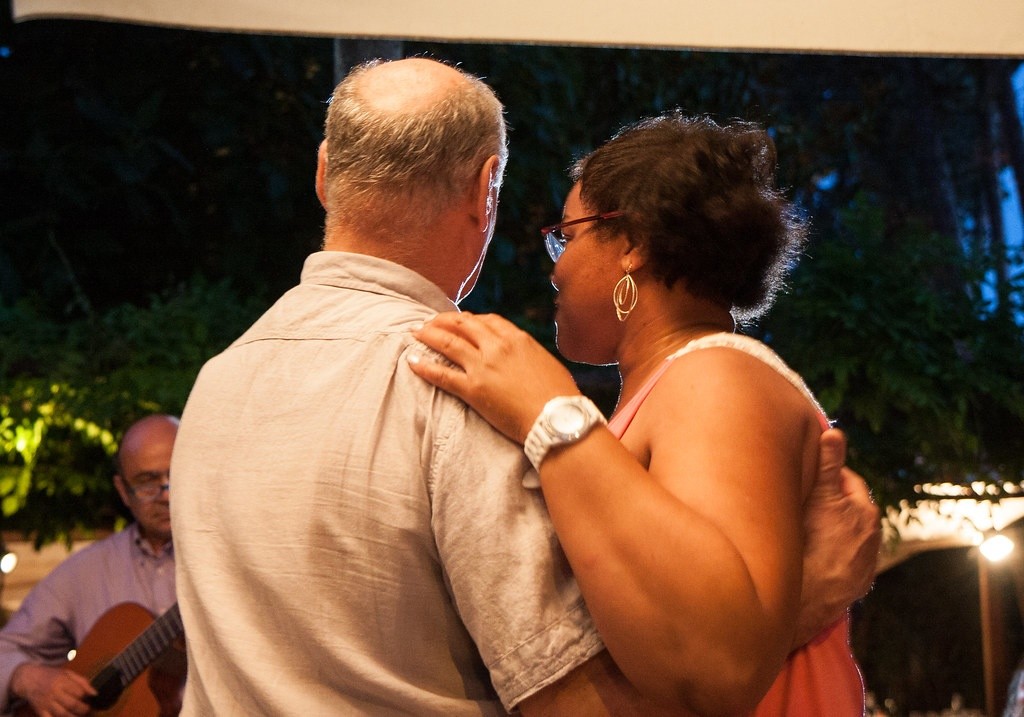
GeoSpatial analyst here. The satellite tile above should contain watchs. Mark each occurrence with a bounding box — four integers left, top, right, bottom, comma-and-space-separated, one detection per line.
522, 397, 608, 489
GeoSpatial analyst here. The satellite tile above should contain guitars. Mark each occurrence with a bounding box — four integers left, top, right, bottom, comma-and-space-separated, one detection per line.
19, 600, 185, 717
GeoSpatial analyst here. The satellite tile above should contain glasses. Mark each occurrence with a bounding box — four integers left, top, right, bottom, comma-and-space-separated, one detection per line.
541, 208, 638, 263
121, 474, 169, 503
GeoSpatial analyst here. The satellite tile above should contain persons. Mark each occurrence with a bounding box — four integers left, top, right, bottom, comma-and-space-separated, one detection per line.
0, 412, 181, 717
409, 106, 870, 717
170, 58, 882, 717
